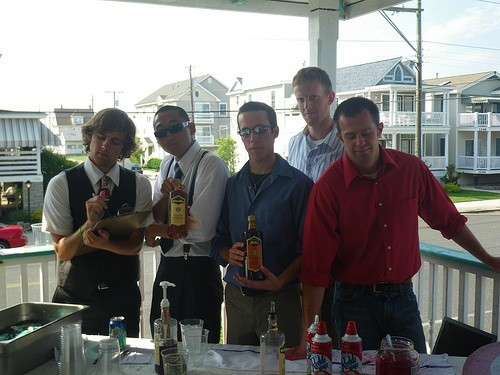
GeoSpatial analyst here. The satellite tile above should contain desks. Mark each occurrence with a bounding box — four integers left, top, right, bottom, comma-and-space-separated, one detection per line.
24, 335, 464, 375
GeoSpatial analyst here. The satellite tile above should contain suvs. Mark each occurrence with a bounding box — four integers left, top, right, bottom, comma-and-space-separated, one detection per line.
131, 164, 143, 174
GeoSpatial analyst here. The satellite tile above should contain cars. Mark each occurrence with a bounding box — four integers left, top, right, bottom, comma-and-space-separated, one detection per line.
0, 222, 28, 249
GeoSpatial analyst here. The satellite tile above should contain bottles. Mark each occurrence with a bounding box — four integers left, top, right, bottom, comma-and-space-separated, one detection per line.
311, 321, 333, 374
241, 215, 264, 280
168, 179, 187, 239
340, 321, 362, 375
259, 301, 285, 375
306, 315, 320, 375
376, 337, 420, 375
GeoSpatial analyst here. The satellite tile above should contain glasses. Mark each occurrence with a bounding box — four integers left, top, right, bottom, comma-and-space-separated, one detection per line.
237, 125, 274, 137
154, 121, 191, 137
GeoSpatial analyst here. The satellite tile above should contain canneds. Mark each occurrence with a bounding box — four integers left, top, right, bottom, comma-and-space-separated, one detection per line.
109, 316, 126, 350
375, 336, 420, 375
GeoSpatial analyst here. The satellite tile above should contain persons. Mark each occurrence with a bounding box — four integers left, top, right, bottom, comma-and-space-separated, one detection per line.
209, 102, 314, 348
285, 97, 500, 360
287, 66, 345, 183
144, 106, 230, 344
41, 107, 153, 338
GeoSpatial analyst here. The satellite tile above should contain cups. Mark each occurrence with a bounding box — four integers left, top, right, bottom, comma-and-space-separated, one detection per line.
179, 319, 204, 350
31, 223, 44, 245
55, 322, 86, 374
183, 328, 209, 367
97, 339, 124, 375
161, 348, 190, 375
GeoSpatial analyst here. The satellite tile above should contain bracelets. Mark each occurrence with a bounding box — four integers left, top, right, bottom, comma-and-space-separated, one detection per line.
78, 228, 83, 237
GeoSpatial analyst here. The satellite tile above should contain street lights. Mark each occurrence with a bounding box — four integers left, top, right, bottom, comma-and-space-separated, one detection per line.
106, 90, 123, 108
25, 180, 32, 223
186, 65, 198, 138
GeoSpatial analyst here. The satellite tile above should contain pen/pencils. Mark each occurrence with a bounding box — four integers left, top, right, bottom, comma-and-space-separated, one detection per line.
362, 361, 371, 365
93, 354, 103, 365
92, 193, 112, 216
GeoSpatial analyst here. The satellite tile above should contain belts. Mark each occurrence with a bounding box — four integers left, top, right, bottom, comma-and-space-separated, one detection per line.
233, 278, 300, 296
330, 280, 412, 293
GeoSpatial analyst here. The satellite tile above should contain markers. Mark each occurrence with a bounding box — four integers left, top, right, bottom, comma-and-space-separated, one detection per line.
112, 350, 129, 361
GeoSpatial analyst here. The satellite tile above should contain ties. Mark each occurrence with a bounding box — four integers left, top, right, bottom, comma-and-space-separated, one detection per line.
101, 176, 110, 193
159, 163, 183, 254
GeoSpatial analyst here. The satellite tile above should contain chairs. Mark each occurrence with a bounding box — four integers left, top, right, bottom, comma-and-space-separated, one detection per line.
430, 317, 497, 358
